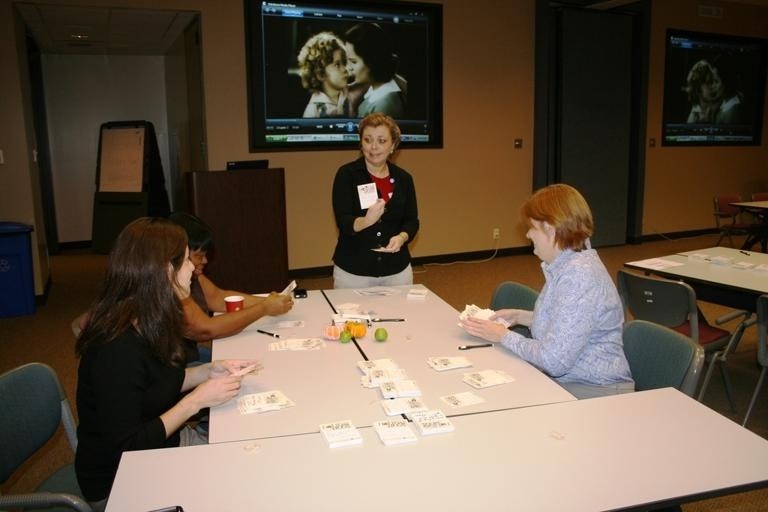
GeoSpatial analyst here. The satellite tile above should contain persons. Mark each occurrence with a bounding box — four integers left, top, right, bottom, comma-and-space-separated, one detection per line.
685, 59, 718, 124
74, 217, 259, 512
296, 31, 350, 119
331, 112, 420, 289
344, 23, 408, 120
711, 54, 745, 124
462, 184, 635, 400
165, 211, 294, 368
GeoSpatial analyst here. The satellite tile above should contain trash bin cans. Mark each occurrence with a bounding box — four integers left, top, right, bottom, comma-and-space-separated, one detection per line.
0, 221, 36, 319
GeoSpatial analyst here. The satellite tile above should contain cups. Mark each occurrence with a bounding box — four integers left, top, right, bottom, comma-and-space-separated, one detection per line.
224, 294, 246, 314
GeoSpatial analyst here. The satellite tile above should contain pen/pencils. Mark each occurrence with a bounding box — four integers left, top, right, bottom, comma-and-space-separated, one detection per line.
459, 344, 494, 350
371, 318, 406, 322
739, 250, 750, 256
257, 330, 282, 338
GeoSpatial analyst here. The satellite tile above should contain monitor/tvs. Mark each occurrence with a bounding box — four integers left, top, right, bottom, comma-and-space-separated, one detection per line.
244, 0, 444, 153
226, 159, 269, 170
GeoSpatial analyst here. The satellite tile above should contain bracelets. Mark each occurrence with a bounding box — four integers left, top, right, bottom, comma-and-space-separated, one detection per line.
400, 234, 406, 242
